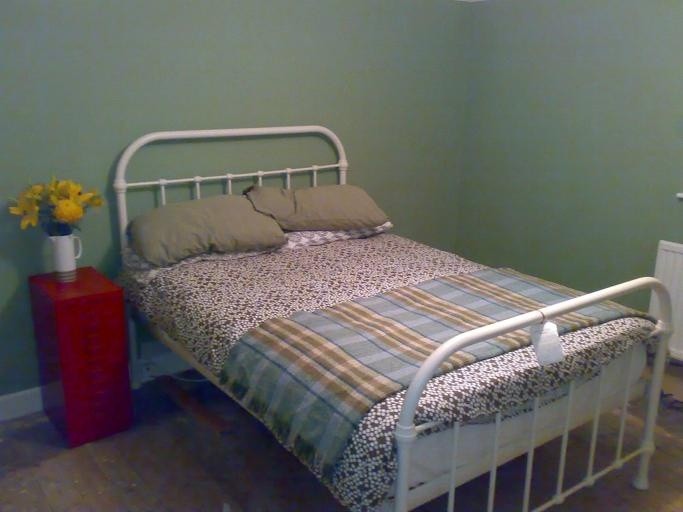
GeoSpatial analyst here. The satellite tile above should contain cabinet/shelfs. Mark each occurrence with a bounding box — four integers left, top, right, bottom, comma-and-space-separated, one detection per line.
642, 238, 682, 363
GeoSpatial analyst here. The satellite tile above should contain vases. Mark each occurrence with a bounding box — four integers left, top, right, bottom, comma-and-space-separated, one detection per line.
46, 232, 83, 282
8, 169, 103, 236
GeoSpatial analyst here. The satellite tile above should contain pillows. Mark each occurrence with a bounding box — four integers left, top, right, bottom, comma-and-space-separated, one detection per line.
246, 182, 389, 230
119, 242, 282, 287
128, 192, 288, 269
282, 219, 393, 250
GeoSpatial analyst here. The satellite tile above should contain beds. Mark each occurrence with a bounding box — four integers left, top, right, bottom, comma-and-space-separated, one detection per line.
112, 126, 673, 510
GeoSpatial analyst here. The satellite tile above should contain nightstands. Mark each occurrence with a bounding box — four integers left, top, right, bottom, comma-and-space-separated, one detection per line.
28, 265, 136, 448
